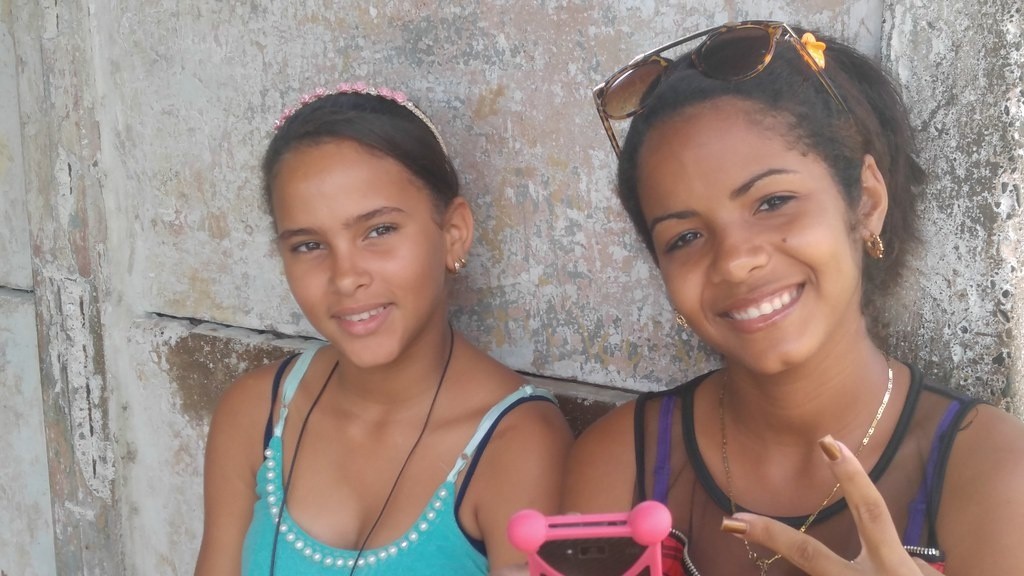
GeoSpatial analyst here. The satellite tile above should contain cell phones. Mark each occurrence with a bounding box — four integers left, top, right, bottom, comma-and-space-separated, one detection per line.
510, 500, 673, 576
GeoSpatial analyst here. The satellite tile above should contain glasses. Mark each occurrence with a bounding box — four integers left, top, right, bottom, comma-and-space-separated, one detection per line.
592, 20, 864, 166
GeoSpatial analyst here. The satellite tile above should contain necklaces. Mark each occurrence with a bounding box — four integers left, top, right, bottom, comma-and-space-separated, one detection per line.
720, 349, 893, 576
270, 321, 454, 576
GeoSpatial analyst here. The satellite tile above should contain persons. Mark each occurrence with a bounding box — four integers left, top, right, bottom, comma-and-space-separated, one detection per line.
193, 83, 576, 576
558, 20, 1024, 576
